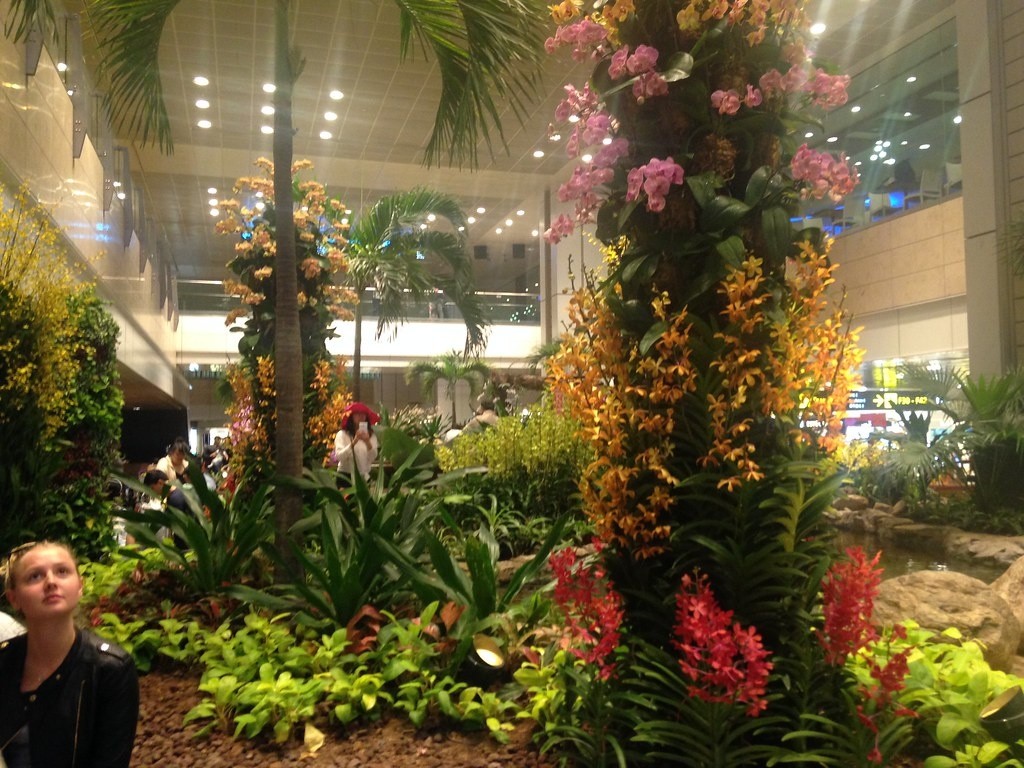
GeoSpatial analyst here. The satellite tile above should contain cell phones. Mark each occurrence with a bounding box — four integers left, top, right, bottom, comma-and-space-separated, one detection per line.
359, 422, 367, 433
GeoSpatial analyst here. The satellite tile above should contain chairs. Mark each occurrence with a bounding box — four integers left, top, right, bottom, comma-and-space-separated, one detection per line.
800, 163, 963, 237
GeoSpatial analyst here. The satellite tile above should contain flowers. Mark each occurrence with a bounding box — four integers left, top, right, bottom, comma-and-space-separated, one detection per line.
538, 0, 862, 252
213, 159, 357, 480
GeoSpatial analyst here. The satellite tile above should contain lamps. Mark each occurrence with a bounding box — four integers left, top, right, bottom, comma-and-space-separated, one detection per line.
454, 634, 503, 699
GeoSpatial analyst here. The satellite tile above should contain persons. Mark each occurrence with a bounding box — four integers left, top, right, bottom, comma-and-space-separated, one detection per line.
109, 397, 502, 550
0, 538, 137, 768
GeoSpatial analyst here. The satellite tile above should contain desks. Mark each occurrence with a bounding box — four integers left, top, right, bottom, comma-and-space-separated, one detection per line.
886, 181, 921, 210
813, 208, 842, 237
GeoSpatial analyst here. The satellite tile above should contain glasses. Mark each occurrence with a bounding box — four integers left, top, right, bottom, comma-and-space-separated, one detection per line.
8, 542, 39, 590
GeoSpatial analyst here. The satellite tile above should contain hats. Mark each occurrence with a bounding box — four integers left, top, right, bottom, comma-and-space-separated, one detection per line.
341, 402, 382, 430
477, 392, 494, 407
